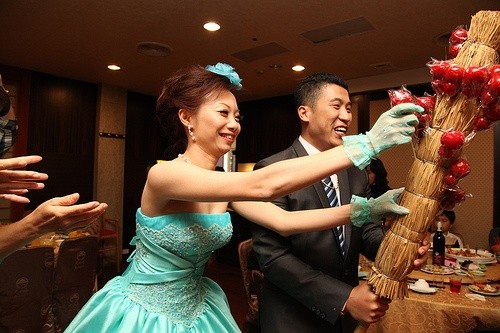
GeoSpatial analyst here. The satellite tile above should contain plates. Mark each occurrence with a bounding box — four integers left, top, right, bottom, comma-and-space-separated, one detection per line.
456, 269, 468, 276
421, 265, 453, 274
478, 264, 488, 272
445, 247, 496, 262
467, 284, 500, 296
408, 285, 437, 294
470, 270, 485, 276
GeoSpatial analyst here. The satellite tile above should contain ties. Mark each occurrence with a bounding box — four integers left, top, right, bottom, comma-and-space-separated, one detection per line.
321, 176, 344, 256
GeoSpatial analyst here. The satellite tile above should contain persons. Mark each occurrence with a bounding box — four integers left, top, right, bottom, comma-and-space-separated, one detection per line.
63, 62, 424, 333
250, 73, 431, 333
430, 210, 463, 248
365, 156, 394, 228
0, 75, 108, 262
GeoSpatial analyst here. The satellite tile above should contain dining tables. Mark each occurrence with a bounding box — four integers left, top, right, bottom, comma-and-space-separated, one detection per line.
358, 257, 500, 333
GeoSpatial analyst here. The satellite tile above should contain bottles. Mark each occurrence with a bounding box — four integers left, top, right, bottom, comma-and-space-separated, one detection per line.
432, 221, 446, 266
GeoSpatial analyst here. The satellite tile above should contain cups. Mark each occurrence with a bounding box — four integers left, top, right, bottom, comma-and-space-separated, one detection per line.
449, 276, 463, 297
432, 270, 444, 291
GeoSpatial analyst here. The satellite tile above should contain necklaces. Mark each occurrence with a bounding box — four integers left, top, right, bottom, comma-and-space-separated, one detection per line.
178, 154, 190, 164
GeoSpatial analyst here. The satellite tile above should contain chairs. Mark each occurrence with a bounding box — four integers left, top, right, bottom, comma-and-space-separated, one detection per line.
96, 213, 121, 264
238, 238, 265, 324
54, 235, 99, 326
0, 244, 56, 333
